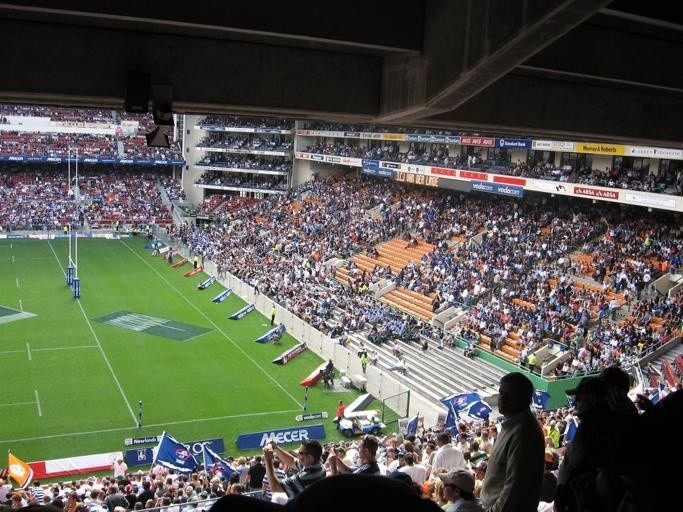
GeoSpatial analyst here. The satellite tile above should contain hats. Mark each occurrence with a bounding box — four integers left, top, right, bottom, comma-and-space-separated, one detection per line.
438, 467, 475, 493
471, 460, 489, 473
206, 471, 445, 511
565, 375, 611, 396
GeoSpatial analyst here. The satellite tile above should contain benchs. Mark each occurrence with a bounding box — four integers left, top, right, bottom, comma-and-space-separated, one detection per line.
0, 102, 296, 237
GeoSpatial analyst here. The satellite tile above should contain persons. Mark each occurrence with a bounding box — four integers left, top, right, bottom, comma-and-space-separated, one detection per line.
1, 403, 683, 510
148, 112, 682, 403
0, 101, 187, 237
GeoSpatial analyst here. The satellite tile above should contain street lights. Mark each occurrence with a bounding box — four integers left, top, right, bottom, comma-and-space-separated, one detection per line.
73, 147, 79, 201
66, 143, 72, 197
